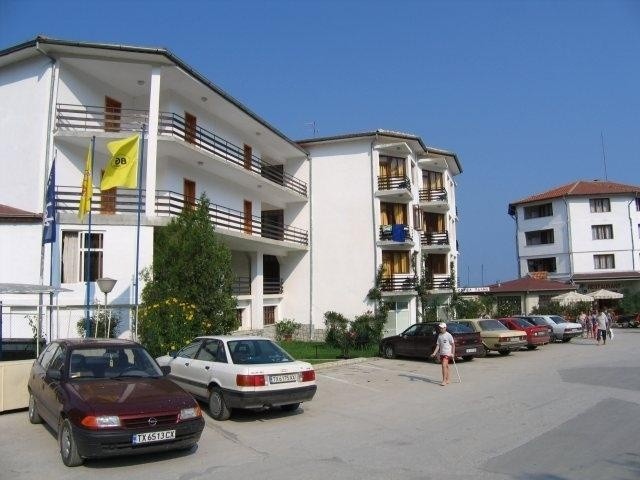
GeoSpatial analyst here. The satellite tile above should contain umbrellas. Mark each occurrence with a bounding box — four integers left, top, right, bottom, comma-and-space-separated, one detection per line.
551, 291, 594, 302
587, 289, 624, 299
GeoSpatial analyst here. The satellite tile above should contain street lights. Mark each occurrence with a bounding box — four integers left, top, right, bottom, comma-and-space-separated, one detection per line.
480, 264, 485, 287
467, 264, 470, 288
96, 276, 117, 337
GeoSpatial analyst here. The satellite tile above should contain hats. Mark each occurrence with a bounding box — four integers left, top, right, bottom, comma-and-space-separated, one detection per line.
439, 322, 447, 328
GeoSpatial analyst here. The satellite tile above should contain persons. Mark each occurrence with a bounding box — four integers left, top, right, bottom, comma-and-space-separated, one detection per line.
576, 308, 612, 345
431, 323, 456, 386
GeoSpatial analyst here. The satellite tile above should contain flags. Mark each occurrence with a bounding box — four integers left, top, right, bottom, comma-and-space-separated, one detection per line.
42, 159, 56, 244
99, 135, 139, 192
79, 143, 93, 225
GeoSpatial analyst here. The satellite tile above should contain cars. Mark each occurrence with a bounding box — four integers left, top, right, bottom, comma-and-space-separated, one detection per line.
494, 317, 551, 352
514, 314, 554, 345
531, 314, 583, 343
452, 318, 528, 357
26, 336, 204, 465
379, 321, 484, 363
154, 336, 318, 421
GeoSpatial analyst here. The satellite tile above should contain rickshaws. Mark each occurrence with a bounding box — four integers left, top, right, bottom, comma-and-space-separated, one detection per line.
612, 313, 637, 329
